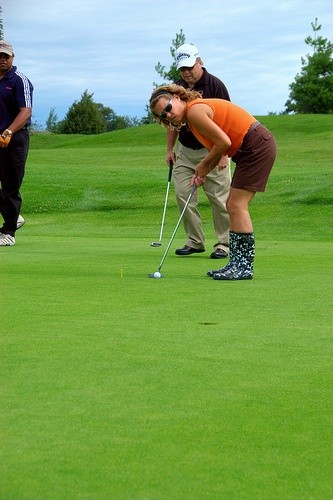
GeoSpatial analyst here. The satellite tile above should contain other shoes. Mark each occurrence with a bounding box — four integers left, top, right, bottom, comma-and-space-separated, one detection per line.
0, 234, 16, 246
209, 248, 229, 259
16, 215, 26, 230
175, 245, 205, 256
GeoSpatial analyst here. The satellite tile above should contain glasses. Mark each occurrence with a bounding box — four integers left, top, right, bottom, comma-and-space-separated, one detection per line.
179, 67, 194, 72
0, 54, 11, 59
159, 96, 174, 121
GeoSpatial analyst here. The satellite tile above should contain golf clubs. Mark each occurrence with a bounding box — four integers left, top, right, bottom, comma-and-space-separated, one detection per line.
150, 158, 174, 246
148, 184, 197, 277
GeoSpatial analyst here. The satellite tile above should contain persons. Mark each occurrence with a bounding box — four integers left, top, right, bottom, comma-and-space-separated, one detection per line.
149, 84, 276, 281
165, 44, 232, 258
0, 41, 34, 245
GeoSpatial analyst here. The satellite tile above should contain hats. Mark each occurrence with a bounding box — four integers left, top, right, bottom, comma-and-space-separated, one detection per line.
174, 43, 200, 72
0, 40, 15, 56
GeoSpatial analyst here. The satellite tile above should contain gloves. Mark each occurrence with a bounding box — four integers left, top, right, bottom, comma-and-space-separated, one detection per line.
0, 129, 12, 148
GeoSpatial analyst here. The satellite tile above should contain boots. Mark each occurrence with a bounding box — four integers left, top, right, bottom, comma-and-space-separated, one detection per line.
211, 232, 256, 280
206, 231, 239, 277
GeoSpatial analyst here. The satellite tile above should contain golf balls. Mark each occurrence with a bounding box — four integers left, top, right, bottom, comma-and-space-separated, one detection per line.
154, 272, 161, 278
